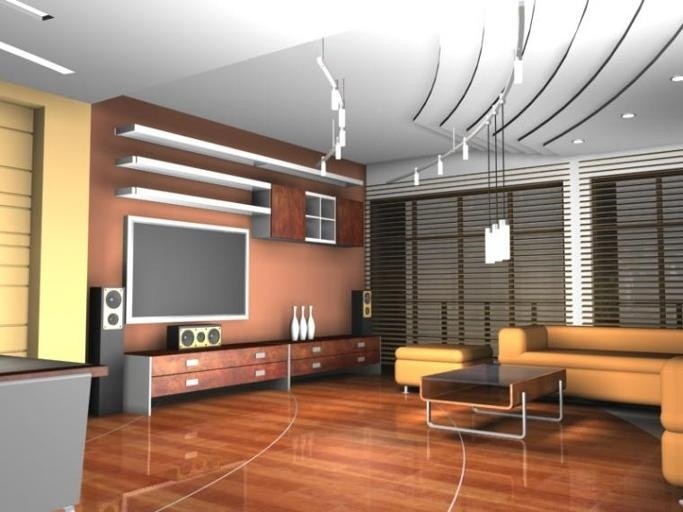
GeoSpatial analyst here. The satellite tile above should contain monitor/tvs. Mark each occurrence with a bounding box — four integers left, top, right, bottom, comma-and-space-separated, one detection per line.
124, 216, 251, 324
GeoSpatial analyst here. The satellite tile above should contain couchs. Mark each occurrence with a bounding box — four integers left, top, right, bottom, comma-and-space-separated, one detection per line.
661, 355, 681, 485
394, 344, 493, 395
497, 326, 683, 403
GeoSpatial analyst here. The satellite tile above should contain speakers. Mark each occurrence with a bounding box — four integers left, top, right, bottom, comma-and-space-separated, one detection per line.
353, 290, 372, 337
166, 325, 224, 349
90, 285, 125, 417
